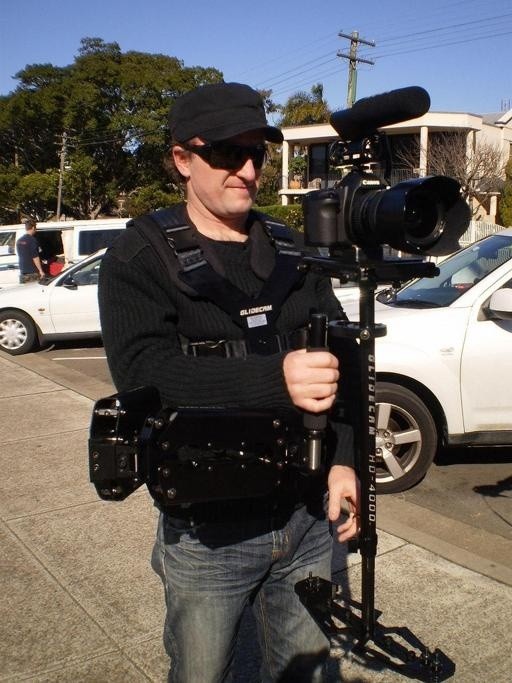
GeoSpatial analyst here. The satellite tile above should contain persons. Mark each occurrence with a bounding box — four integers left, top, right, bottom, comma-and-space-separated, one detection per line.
98, 83, 361, 683
16, 220, 45, 283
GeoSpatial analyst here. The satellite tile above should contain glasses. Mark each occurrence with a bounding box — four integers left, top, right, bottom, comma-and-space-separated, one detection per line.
183, 143, 267, 169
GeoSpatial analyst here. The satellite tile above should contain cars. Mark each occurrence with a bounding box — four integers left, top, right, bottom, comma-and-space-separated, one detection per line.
0, 247, 109, 355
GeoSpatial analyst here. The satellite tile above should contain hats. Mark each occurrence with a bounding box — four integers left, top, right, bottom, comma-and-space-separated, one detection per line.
169, 83, 284, 143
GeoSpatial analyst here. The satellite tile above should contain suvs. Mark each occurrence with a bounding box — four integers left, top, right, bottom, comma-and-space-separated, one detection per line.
332, 226, 512, 496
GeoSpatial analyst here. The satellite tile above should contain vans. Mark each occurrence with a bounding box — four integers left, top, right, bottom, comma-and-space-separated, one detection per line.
0, 218, 133, 286
